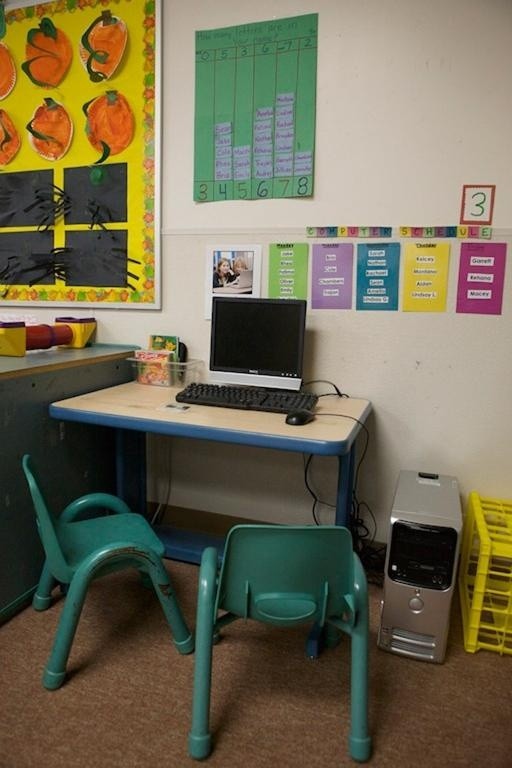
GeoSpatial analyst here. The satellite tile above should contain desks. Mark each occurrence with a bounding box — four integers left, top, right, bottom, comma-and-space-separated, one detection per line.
52, 379, 372, 656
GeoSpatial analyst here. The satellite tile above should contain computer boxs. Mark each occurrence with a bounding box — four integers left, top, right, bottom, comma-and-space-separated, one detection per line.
376, 469, 463, 664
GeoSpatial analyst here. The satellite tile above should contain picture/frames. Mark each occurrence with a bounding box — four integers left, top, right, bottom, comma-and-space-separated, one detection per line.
204, 244, 262, 319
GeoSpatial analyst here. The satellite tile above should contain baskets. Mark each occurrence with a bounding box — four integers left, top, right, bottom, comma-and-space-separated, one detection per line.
461, 488, 511, 658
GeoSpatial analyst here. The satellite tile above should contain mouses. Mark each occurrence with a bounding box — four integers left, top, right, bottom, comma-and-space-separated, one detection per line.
285, 409, 314, 425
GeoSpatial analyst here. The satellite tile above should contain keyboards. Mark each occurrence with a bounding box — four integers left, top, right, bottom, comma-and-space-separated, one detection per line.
175, 382, 319, 413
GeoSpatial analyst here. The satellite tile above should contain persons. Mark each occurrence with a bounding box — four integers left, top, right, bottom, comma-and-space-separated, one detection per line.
213, 257, 236, 289
233, 257, 249, 277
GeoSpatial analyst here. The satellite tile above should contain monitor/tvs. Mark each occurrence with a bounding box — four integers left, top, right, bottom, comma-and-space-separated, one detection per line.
207, 293, 307, 391
